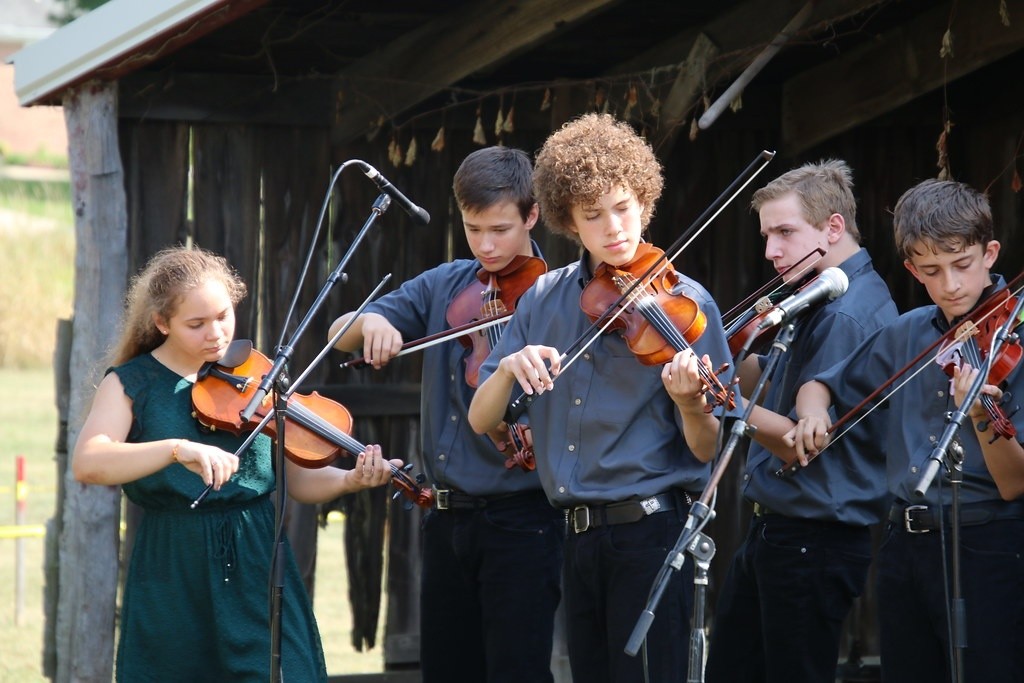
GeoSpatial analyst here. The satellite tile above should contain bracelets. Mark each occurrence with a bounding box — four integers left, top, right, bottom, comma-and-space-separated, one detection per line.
173, 436, 188, 462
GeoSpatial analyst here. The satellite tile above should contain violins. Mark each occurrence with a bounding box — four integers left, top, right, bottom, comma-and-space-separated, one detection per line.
184, 340, 454, 518
930, 278, 1024, 446
441, 245, 559, 478
572, 235, 750, 427
719, 264, 834, 369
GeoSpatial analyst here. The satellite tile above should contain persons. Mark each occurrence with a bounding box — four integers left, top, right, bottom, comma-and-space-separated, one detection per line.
465, 114, 744, 681
327, 146, 562, 683
784, 177, 1024, 683
69, 245, 406, 683
708, 160, 897, 683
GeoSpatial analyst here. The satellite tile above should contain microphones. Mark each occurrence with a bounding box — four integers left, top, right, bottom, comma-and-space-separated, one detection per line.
758, 267, 849, 329
357, 161, 430, 226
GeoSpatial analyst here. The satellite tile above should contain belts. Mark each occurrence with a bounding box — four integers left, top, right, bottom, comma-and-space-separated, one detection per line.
565, 494, 675, 533
754, 503, 779, 514
889, 502, 1024, 533
436, 489, 517, 510
